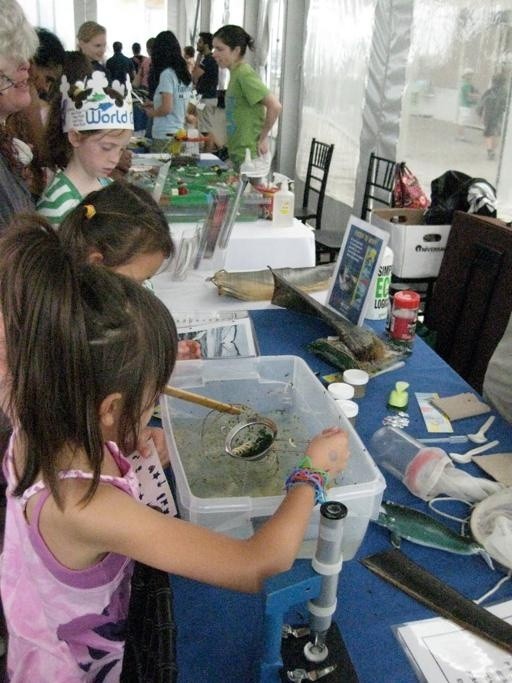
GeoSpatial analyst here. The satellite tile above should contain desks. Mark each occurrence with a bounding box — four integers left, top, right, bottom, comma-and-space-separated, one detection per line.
126, 261, 511, 682
116, 147, 319, 285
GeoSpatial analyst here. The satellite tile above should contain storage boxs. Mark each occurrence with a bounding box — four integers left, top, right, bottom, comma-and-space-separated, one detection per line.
153, 348, 388, 568
366, 204, 455, 283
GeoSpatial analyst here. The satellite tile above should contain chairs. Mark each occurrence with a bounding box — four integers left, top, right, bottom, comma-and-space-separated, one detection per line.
383, 169, 499, 328
291, 136, 337, 233
311, 150, 407, 268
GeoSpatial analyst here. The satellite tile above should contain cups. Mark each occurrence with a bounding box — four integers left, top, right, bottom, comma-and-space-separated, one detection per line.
328, 367, 369, 428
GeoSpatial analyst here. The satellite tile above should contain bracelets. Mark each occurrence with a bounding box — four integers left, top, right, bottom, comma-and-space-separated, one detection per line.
282, 456, 330, 508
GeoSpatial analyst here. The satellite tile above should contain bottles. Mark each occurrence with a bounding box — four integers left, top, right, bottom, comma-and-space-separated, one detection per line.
167, 127, 199, 154
370, 425, 444, 497
273, 175, 294, 228
390, 288, 416, 350
364, 247, 395, 321
240, 147, 256, 174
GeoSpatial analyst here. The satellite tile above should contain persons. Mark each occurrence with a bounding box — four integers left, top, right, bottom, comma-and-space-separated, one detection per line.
0, 224, 351, 683
454, 68, 481, 142
1, 180, 175, 436
475, 73, 508, 160
